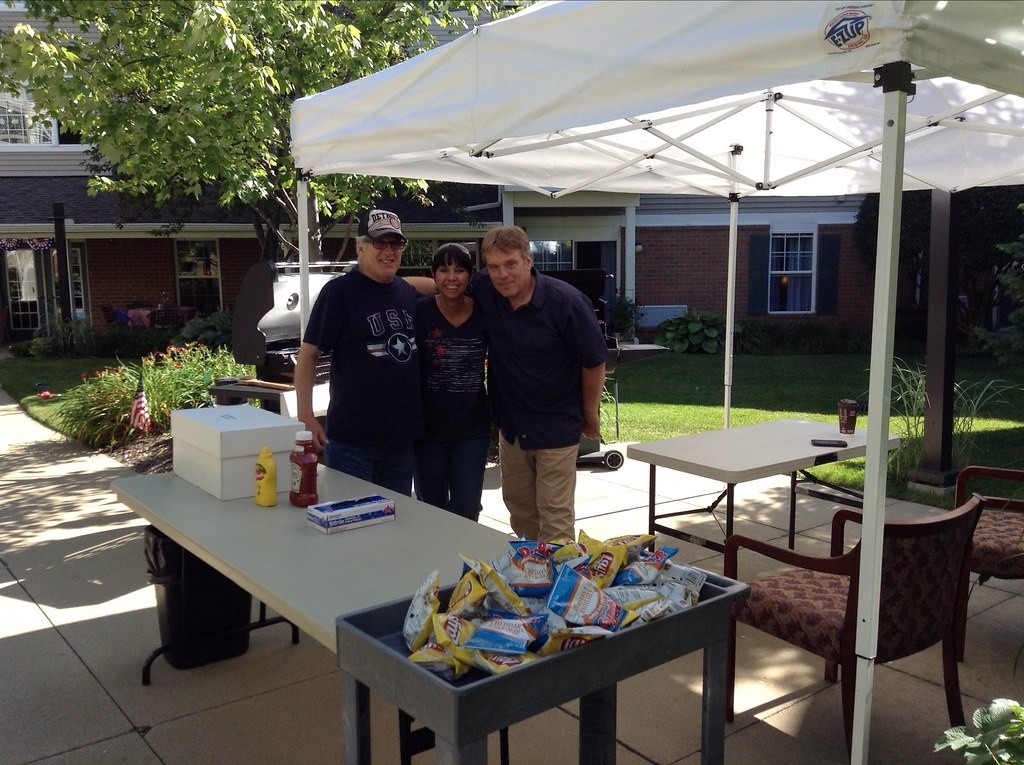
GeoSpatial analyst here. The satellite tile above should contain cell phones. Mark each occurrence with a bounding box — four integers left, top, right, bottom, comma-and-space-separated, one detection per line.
811, 439, 848, 447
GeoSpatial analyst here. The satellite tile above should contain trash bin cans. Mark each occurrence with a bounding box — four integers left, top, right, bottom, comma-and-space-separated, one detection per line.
144, 524, 254, 671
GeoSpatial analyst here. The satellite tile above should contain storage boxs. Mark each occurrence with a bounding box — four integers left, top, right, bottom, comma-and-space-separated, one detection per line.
170, 405, 306, 502
306, 495, 395, 536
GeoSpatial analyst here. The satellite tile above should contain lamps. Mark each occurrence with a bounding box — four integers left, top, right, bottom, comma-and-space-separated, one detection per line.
635, 239, 643, 254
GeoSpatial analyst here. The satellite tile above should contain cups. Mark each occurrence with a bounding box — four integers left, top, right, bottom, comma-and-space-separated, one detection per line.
838, 399, 859, 434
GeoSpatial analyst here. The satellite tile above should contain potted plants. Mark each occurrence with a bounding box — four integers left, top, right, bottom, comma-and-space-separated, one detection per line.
606, 296, 641, 342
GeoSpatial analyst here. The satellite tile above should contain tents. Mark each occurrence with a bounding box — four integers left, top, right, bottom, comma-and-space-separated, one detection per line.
292, 1, 1024, 765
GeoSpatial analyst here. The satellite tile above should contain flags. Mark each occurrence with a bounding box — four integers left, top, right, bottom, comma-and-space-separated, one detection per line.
130, 374, 152, 433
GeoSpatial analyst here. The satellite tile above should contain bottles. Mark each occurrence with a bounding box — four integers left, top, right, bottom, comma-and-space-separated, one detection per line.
289, 431, 318, 506
256, 447, 276, 506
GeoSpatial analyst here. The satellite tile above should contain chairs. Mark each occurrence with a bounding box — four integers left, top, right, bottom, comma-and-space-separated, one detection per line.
100, 303, 128, 328
956, 465, 1024, 581
723, 492, 986, 765
132, 300, 153, 307
157, 308, 178, 335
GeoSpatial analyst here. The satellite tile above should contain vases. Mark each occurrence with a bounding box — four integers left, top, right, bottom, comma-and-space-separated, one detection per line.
162, 298, 171, 312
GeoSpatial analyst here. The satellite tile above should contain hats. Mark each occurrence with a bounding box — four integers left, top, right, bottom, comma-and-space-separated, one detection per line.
358, 210, 407, 240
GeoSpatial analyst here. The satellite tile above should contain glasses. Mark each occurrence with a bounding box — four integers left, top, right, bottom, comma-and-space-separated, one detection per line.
432, 243, 471, 260
360, 237, 408, 251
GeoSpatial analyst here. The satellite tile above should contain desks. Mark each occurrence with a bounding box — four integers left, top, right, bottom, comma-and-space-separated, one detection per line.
127, 307, 196, 330
626, 418, 901, 554
109, 464, 520, 765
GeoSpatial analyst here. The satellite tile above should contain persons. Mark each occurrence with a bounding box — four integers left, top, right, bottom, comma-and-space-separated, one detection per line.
293, 209, 609, 544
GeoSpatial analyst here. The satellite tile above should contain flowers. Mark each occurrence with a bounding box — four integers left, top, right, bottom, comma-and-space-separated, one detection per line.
160, 290, 169, 298
